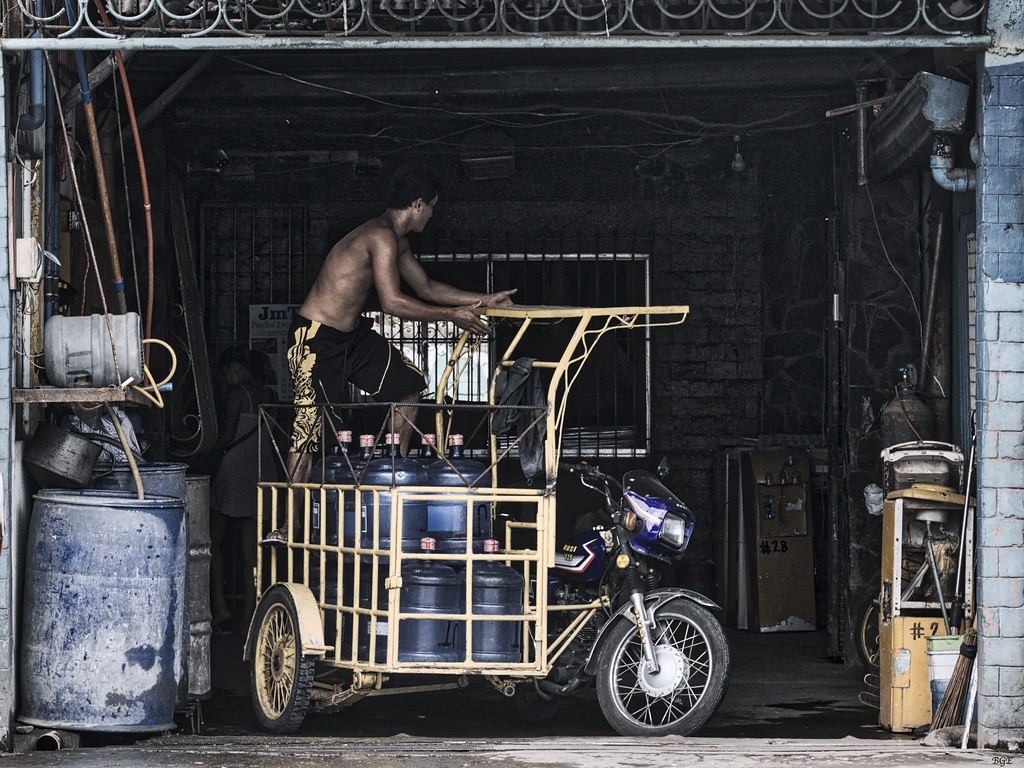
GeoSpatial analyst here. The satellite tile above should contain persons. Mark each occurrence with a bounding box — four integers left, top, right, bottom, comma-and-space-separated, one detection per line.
261, 169, 519, 540
200, 345, 286, 637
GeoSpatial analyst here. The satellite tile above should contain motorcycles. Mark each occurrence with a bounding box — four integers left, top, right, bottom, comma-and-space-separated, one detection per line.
545, 461, 732, 737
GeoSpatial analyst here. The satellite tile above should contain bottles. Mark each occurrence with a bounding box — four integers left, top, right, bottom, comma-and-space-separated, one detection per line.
325, 536, 525, 663
307, 429, 491, 565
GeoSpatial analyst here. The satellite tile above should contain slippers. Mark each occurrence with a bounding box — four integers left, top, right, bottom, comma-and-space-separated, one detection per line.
258, 530, 297, 554
211, 622, 233, 637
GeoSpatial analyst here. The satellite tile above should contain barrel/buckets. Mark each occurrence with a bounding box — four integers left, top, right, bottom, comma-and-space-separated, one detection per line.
923, 650, 978, 727
23, 420, 115, 486
44, 311, 143, 387
15, 460, 212, 733
